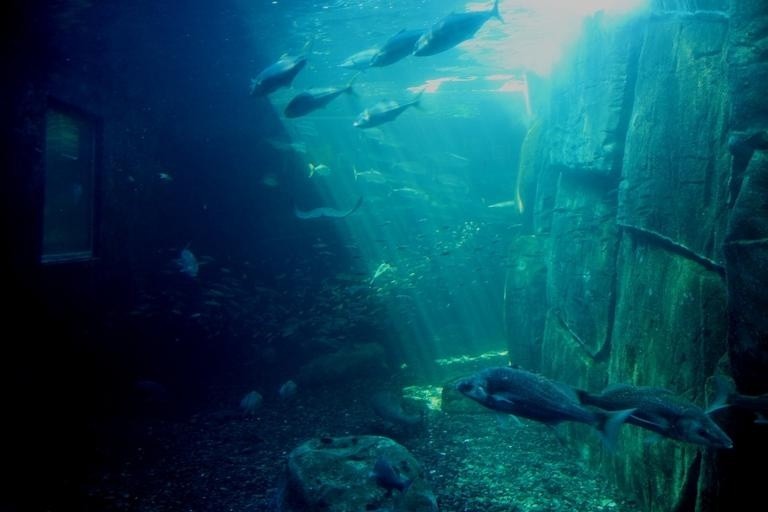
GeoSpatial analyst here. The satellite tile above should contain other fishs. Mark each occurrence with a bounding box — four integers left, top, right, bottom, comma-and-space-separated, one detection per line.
120, 162, 517, 350
